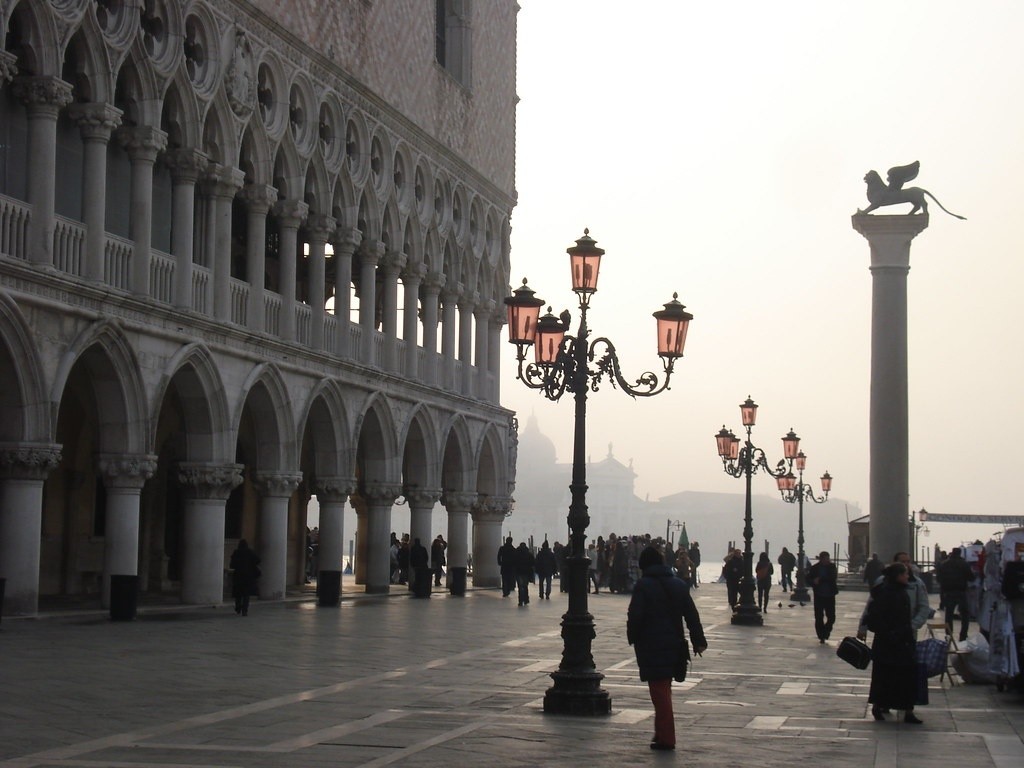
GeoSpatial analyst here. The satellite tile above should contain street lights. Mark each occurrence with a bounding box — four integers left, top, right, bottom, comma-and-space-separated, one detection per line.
911, 508, 930, 563
713, 393, 786, 626
776, 424, 833, 601
503, 226, 694, 717
665, 519, 684, 542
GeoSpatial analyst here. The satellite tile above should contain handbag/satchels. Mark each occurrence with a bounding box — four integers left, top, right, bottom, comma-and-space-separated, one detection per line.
837, 636, 872, 670
672, 638, 690, 682
248, 565, 261, 580
913, 637, 949, 678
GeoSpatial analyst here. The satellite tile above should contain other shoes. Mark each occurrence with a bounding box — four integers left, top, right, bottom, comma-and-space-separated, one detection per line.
235, 608, 240, 614
904, 713, 923, 723
872, 706, 885, 721
242, 610, 247, 617
650, 741, 674, 749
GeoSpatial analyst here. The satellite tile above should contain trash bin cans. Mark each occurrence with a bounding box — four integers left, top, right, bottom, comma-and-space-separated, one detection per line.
452, 567, 467, 596
317, 570, 342, 607
109, 574, 140, 619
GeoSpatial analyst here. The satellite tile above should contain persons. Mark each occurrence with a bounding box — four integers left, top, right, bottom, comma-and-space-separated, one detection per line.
863, 552, 885, 587
940, 548, 975, 642
410, 538, 428, 599
855, 551, 931, 640
809, 552, 838, 644
778, 547, 796, 592
305, 527, 319, 579
723, 548, 743, 612
867, 562, 922, 724
935, 550, 947, 610
390, 532, 409, 585
626, 547, 708, 749
230, 539, 261, 616
497, 536, 567, 606
587, 533, 701, 594
756, 553, 773, 614
431, 534, 447, 586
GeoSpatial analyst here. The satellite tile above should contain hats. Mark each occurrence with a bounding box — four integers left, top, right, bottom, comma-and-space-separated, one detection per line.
542, 540, 548, 548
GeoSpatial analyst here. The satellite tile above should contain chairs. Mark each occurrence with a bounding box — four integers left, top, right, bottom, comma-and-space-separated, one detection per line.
928, 623, 971, 686
